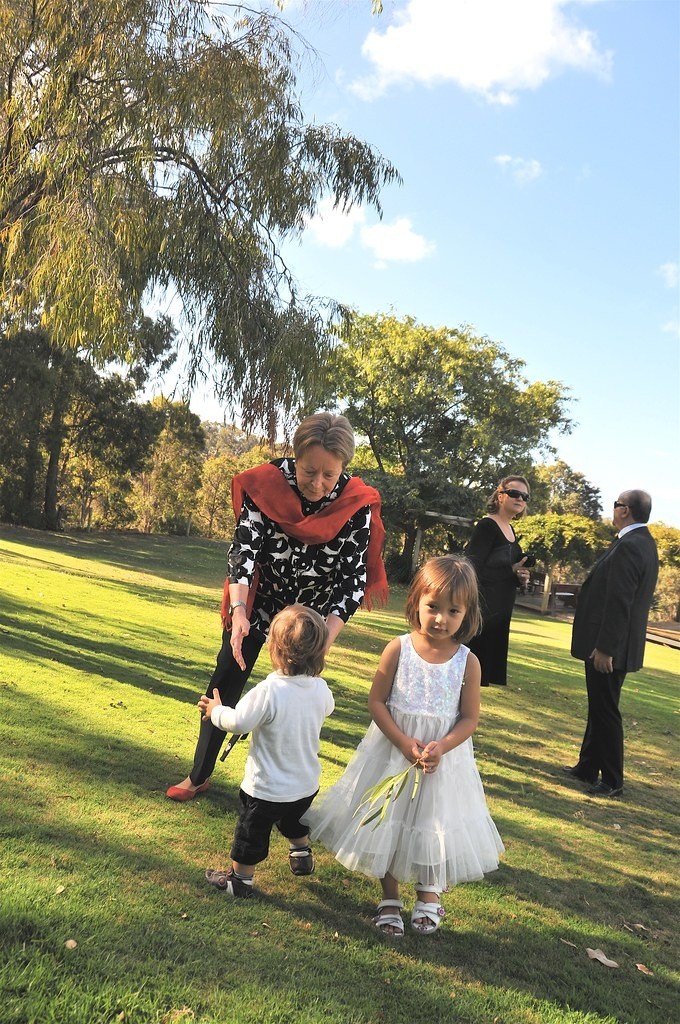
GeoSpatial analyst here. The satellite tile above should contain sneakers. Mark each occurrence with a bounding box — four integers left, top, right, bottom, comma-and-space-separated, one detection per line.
288, 847, 314, 875
205, 863, 254, 899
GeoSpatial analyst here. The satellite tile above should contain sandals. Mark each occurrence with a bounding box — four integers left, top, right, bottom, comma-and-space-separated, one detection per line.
376, 899, 404, 938
410, 882, 444, 935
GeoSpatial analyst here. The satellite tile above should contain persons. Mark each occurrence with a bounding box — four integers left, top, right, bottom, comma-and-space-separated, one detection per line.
197, 606, 335, 897
301, 553, 505, 936
563, 490, 659, 799
463, 476, 530, 687
168, 413, 384, 802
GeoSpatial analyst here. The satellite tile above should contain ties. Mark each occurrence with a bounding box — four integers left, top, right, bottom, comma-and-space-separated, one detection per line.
612, 535, 618, 545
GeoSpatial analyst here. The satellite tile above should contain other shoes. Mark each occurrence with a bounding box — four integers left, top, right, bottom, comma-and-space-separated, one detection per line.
167, 772, 210, 801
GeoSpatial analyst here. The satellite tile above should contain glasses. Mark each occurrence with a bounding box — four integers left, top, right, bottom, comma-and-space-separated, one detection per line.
613, 501, 630, 508
500, 489, 530, 502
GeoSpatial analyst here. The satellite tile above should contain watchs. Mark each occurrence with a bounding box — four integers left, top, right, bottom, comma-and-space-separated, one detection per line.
229, 601, 246, 616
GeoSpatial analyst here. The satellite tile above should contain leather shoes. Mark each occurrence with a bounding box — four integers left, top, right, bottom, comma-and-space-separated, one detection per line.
563, 765, 598, 782
587, 777, 623, 797
